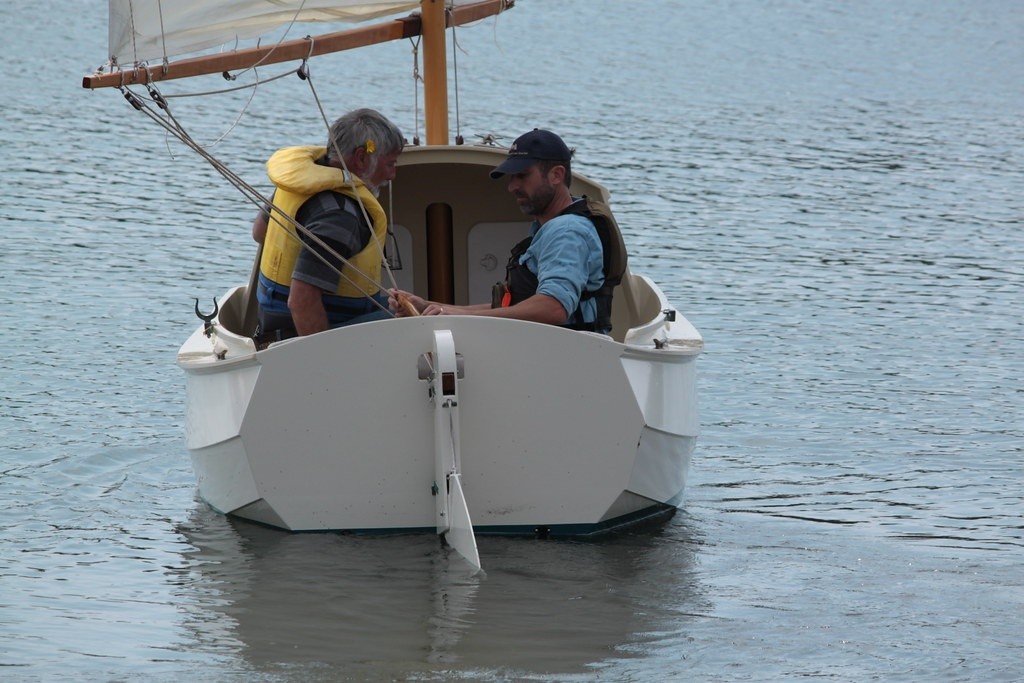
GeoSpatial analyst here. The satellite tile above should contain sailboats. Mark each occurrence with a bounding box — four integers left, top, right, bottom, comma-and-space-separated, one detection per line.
82, 1, 704, 575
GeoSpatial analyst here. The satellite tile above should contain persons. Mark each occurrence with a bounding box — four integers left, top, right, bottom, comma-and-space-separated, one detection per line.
252, 107, 409, 342
388, 124, 620, 355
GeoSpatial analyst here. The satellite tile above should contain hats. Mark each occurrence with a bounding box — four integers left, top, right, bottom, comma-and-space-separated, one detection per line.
489, 127, 571, 179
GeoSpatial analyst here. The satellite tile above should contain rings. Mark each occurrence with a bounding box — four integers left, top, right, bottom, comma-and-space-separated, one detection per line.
440, 307, 443, 314
